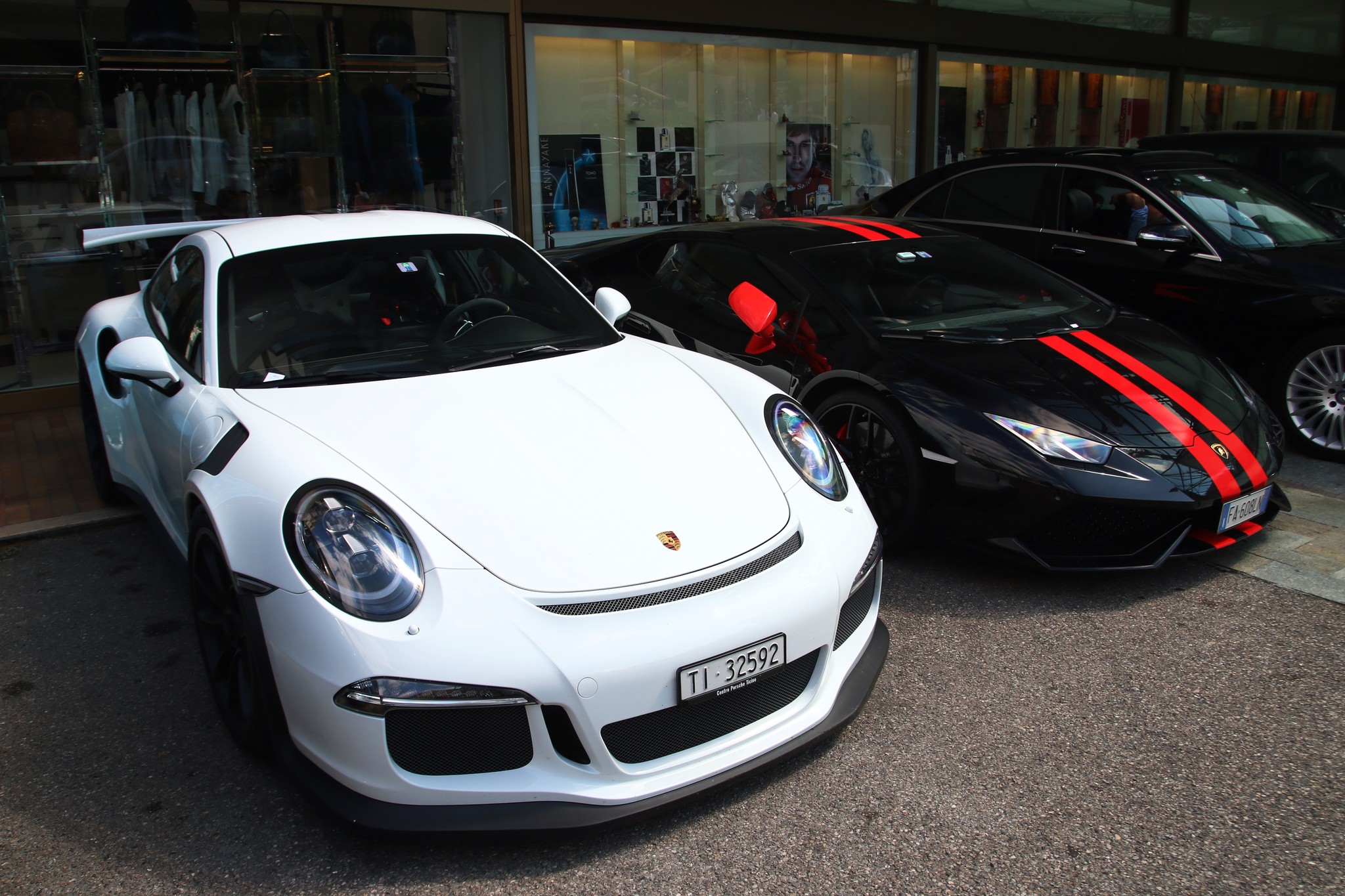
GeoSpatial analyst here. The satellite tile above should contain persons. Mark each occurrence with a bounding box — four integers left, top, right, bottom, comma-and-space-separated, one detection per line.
719, 180, 778, 223
786, 123, 832, 215
855, 127, 886, 205
1108, 187, 1184, 243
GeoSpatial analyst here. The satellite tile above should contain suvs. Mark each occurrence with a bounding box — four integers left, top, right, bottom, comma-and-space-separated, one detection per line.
1142, 127, 1345, 238
66, 135, 233, 205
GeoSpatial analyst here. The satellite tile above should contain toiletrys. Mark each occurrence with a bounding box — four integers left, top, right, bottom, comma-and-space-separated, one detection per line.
816, 185, 831, 209
660, 128, 671, 151
641, 202, 653, 223
638, 153, 651, 176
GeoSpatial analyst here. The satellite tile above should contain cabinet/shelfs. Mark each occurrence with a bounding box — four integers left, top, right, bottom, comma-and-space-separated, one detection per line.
238, 52, 335, 161
625, 118, 648, 196
704, 119, 726, 190
843, 122, 859, 186
777, 120, 796, 189
1, 63, 123, 388
91, 48, 242, 271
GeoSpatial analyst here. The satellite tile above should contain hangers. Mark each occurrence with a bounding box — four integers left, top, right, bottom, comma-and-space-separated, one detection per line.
114, 63, 244, 115
339, 64, 459, 97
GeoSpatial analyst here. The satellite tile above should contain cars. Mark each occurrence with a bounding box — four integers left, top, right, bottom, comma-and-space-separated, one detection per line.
821, 144, 1345, 465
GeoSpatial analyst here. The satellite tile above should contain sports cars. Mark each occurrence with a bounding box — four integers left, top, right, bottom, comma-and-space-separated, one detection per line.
72, 208, 890, 831
535, 214, 1294, 577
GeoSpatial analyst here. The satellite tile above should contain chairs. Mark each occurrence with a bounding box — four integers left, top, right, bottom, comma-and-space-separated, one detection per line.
356, 280, 443, 348
219, 288, 337, 373
1066, 191, 1098, 234
1112, 194, 1132, 238
